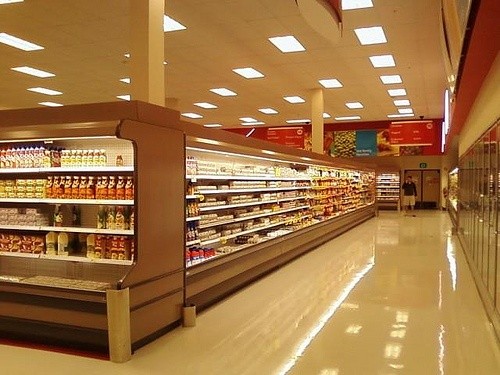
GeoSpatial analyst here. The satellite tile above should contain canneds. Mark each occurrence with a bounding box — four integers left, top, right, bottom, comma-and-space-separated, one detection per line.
187, 247, 215, 266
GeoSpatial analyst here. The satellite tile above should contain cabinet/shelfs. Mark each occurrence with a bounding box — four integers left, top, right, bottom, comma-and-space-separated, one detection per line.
0, 165, 402, 266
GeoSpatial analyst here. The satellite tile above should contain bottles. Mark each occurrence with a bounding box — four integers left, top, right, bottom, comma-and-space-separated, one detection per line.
186, 222, 200, 242
187, 155, 197, 176
115, 155, 124, 166
96, 205, 134, 230
45, 231, 130, 260
49, 204, 81, 227
186, 179, 194, 195
185, 200, 198, 218
46, 175, 135, 201
187, 247, 215, 267
0, 147, 108, 166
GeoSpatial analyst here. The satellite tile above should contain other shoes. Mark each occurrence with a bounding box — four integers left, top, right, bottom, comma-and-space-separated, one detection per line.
412, 214, 416, 217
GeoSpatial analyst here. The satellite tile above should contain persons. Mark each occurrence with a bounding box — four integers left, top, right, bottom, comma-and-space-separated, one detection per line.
402, 175, 417, 217
